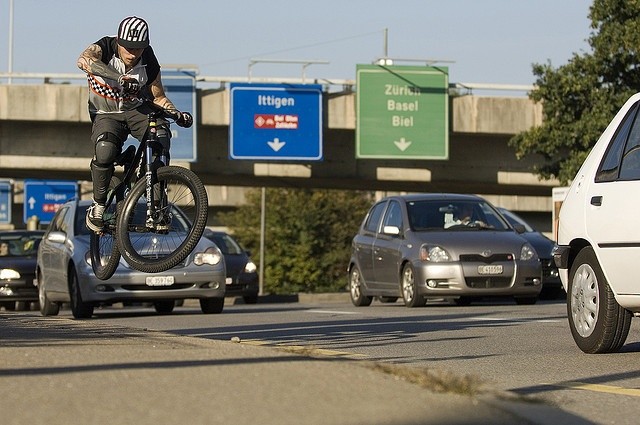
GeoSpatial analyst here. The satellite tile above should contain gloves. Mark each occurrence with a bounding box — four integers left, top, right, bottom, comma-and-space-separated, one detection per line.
176, 112, 193, 128
118, 74, 140, 94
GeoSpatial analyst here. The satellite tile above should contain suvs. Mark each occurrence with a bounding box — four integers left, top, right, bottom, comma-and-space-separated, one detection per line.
552, 92, 640, 353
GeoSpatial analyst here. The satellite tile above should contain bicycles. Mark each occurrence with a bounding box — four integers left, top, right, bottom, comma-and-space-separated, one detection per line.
90, 80, 208, 280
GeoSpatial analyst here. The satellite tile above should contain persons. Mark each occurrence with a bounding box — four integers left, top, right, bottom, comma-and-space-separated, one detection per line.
0, 242, 10, 256
455, 203, 493, 228
77, 16, 193, 231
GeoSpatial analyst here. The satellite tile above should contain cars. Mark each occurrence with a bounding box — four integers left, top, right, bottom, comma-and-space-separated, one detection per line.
496, 208, 563, 300
202, 229, 259, 303
35, 197, 226, 318
0, 230, 45, 311
347, 194, 543, 306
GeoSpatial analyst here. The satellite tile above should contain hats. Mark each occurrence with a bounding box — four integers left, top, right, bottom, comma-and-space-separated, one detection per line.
118, 16, 149, 49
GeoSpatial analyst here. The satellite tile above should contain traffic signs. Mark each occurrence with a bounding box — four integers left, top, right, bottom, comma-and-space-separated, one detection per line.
118, 70, 197, 162
357, 66, 449, 159
230, 84, 322, 160
24, 179, 78, 224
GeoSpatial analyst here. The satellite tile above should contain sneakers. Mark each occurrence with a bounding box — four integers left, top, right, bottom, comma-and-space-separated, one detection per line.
86, 202, 106, 232
145, 212, 173, 230
